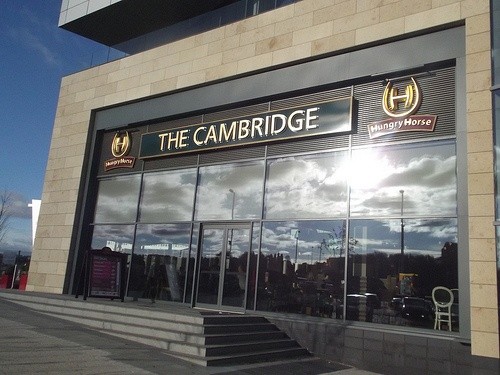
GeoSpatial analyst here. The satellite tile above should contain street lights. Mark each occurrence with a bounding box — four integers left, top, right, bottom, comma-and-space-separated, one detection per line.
399, 189, 405, 275
227, 189, 236, 256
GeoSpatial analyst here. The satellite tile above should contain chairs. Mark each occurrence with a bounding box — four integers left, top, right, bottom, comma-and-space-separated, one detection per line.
432, 287, 458, 332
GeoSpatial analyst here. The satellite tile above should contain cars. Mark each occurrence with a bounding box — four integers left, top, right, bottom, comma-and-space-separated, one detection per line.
400, 295, 430, 322
432, 301, 459, 331
